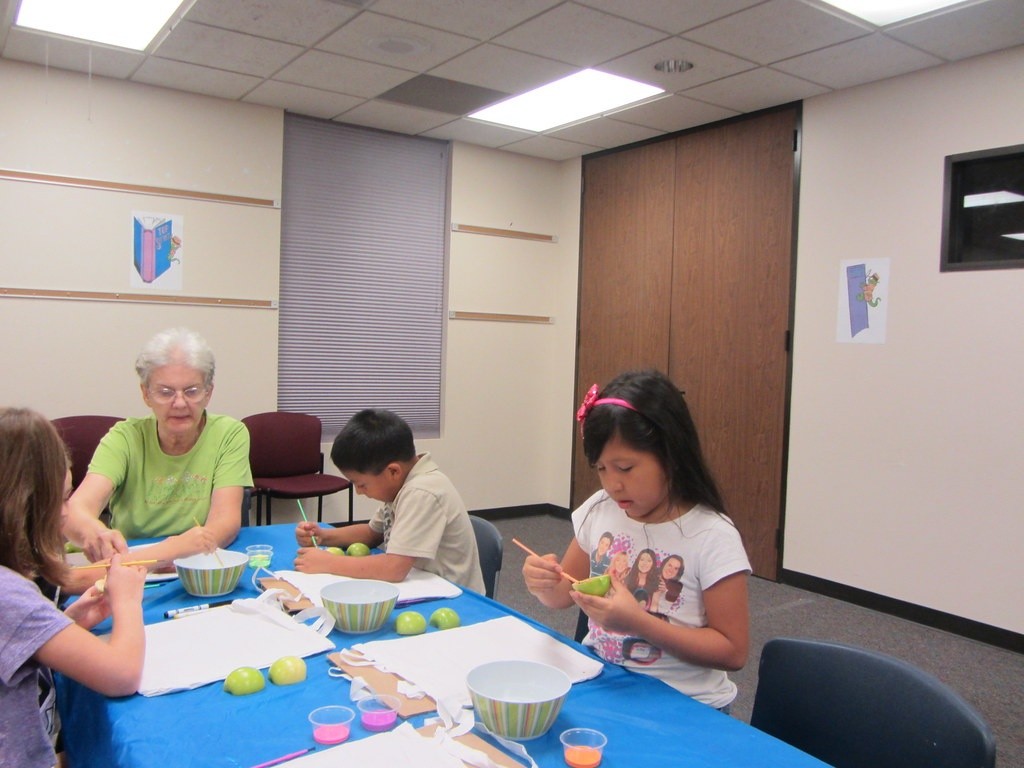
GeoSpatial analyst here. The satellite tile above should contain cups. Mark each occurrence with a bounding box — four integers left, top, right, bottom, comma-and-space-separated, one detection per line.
246, 544, 274, 569
308, 705, 355, 744
559, 727, 607, 768
357, 695, 402, 731
571, 575, 611, 597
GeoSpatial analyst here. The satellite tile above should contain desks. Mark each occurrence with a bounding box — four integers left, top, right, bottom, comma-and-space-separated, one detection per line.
52, 520, 833, 768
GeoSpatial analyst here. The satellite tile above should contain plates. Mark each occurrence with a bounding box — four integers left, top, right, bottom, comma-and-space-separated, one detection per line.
125, 542, 219, 580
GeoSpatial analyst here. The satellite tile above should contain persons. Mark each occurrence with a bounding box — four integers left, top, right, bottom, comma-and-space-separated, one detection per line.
0, 405, 147, 768
29, 467, 217, 768
522, 367, 753, 715
295, 406, 487, 598
61, 325, 255, 573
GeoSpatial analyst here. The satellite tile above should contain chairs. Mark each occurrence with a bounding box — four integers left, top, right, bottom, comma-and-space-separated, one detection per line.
50, 411, 353, 528
469, 515, 588, 644
749, 638, 998, 768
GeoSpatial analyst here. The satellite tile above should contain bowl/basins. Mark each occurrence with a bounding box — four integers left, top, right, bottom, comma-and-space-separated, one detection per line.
465, 658, 573, 741
173, 549, 248, 597
320, 579, 400, 634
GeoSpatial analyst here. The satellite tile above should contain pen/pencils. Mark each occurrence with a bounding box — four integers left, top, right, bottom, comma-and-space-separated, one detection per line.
164, 600, 233, 618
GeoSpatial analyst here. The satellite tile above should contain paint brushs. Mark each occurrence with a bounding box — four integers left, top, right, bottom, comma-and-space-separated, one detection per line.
512, 538, 580, 582
67, 558, 166, 570
251, 745, 316, 768
143, 582, 166, 588
297, 499, 319, 549
195, 518, 224, 564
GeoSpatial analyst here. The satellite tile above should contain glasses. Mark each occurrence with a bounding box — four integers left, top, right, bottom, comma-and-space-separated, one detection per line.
147, 382, 207, 405
